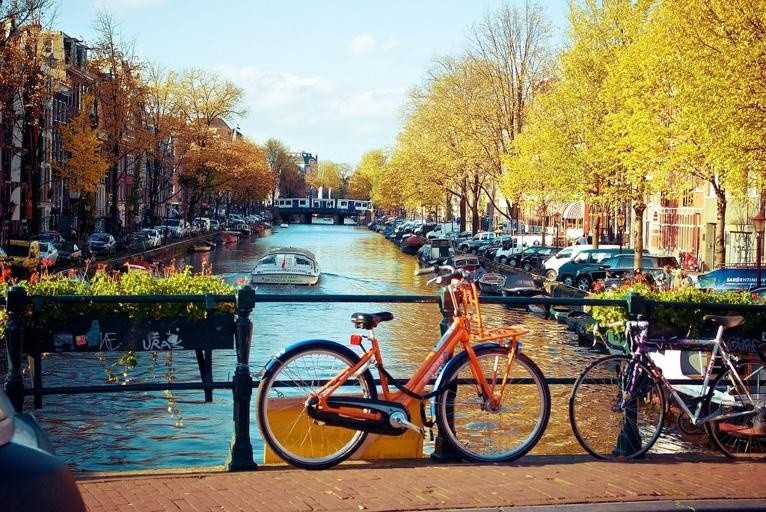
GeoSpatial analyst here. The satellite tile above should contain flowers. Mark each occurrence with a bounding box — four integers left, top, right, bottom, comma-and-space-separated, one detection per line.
583, 272, 764, 327
0, 253, 252, 318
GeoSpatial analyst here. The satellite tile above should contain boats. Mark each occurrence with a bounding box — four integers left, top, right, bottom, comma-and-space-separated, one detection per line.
417, 239, 486, 284
367, 220, 423, 255
251, 246, 320, 285
280, 224, 288, 228
479, 273, 549, 309
597, 322, 765, 436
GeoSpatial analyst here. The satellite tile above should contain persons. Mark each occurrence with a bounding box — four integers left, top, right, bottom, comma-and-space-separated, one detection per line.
578, 235, 588, 245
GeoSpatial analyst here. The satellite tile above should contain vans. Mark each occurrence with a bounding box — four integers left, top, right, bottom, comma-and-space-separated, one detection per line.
541, 245, 677, 292
1, 239, 40, 278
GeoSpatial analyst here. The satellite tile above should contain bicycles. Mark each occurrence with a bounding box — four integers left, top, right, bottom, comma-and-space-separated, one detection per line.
250, 266, 551, 470
569, 312, 766, 461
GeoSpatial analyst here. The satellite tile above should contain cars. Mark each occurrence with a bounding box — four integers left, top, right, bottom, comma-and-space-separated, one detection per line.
28, 231, 82, 270
126, 219, 190, 248
380, 215, 445, 239
445, 230, 563, 272
193, 210, 263, 233
88, 233, 116, 256
